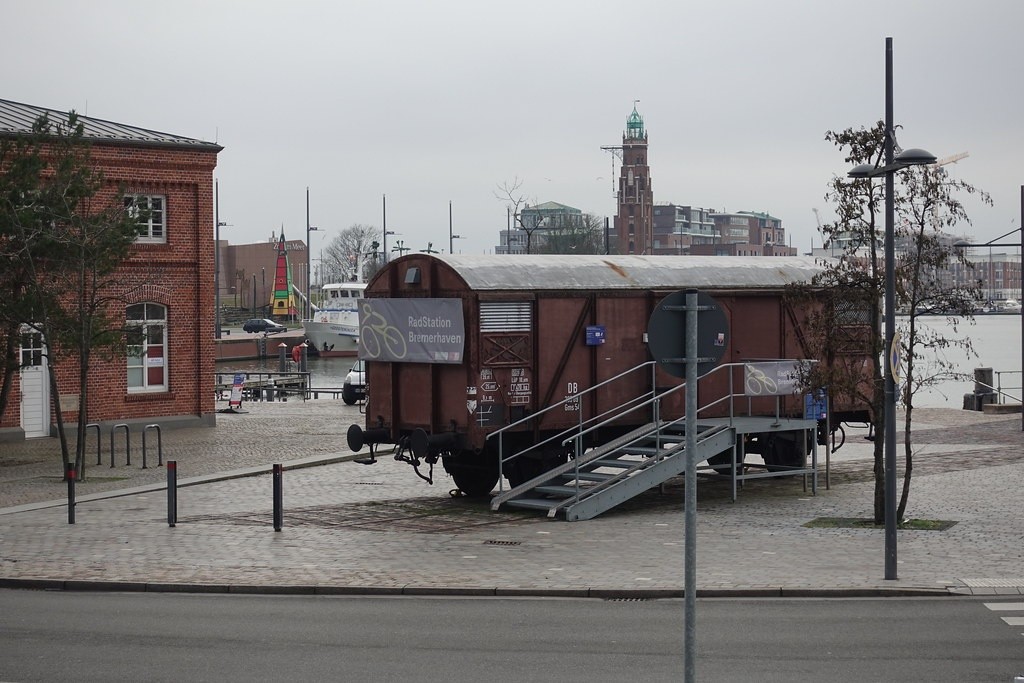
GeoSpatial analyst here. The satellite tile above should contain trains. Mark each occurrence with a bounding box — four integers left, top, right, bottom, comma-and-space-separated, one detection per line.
343, 252, 884, 524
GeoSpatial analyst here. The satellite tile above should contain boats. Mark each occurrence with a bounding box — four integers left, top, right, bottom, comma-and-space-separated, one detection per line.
300, 249, 369, 358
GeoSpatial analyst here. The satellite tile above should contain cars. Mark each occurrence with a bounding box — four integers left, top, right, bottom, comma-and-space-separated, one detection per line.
243, 317, 286, 334
342, 360, 366, 407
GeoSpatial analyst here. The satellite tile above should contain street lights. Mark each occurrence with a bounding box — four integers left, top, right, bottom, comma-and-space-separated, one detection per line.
214, 220, 235, 340
449, 235, 460, 255
848, 148, 941, 582
382, 230, 395, 266
306, 227, 327, 321
